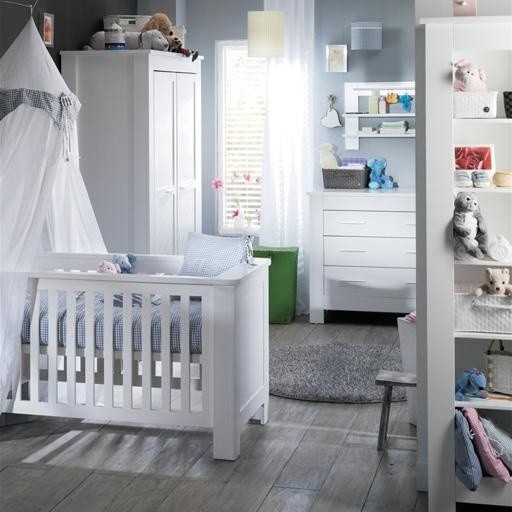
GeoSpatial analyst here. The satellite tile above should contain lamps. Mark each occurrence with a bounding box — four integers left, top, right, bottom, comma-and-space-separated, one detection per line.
350, 22, 383, 51
246, 0, 285, 60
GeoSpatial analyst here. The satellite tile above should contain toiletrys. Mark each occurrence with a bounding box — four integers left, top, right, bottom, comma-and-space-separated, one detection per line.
378, 96, 388, 115
367, 91, 378, 114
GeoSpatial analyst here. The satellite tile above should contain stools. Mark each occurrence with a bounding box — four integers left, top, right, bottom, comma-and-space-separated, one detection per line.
375, 369, 417, 450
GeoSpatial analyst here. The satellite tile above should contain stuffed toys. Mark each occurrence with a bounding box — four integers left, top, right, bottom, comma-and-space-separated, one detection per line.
472, 266, 512, 296
81, 12, 198, 63
97, 261, 123, 275
453, 368, 488, 401
113, 252, 137, 274
452, 189, 492, 260
366, 158, 394, 190
452, 57, 484, 93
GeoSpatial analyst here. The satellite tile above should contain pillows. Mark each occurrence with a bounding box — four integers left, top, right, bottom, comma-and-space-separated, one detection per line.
174, 232, 251, 298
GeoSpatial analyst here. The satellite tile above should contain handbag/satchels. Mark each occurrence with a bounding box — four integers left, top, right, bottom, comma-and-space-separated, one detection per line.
485, 337, 512, 397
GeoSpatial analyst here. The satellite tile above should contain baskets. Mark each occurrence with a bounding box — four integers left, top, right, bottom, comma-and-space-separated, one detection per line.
454, 91, 498, 118
322, 167, 370, 188
454, 284, 512, 333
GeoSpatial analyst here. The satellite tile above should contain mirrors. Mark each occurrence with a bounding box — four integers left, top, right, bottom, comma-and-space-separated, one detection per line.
320, 108, 343, 128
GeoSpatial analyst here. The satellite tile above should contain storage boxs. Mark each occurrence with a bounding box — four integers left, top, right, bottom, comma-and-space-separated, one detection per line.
103, 14, 152, 44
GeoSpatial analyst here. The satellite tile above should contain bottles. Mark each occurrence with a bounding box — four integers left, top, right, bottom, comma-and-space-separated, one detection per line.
379, 97, 386, 113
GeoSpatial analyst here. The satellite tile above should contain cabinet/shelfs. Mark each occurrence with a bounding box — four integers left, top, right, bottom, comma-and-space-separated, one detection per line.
59, 50, 205, 255
419, 16, 512, 512
307, 186, 417, 324
342, 82, 415, 150
251, 246, 299, 324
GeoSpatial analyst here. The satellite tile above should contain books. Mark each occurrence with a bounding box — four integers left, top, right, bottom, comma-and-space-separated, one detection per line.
363, 120, 415, 136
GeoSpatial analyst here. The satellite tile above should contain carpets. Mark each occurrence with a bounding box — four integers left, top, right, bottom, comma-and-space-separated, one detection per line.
264, 340, 408, 403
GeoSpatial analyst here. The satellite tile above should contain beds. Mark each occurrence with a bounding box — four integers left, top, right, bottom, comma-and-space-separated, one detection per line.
0, 250, 271, 462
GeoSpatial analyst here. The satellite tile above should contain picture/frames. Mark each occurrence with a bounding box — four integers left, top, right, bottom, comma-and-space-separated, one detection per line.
454, 145, 495, 189
326, 45, 348, 72
38, 11, 55, 48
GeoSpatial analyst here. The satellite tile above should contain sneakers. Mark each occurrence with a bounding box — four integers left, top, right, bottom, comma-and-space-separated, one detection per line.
456, 171, 490, 187
